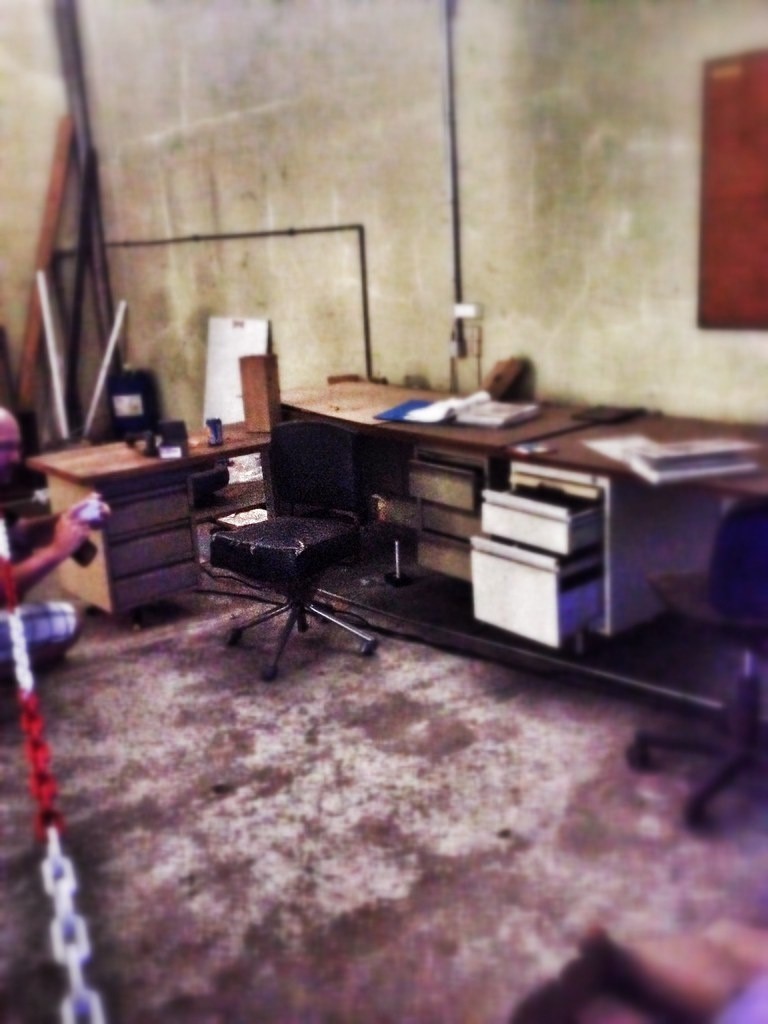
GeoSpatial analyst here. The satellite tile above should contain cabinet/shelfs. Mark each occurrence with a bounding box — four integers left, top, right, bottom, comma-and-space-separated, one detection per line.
26, 422, 272, 627
282, 380, 596, 588
470, 413, 767, 653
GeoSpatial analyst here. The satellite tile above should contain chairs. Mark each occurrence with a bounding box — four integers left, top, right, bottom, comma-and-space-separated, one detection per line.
209, 419, 378, 682
626, 495, 767, 824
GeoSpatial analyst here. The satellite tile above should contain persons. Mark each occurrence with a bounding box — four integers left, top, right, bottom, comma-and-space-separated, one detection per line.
0, 406, 111, 682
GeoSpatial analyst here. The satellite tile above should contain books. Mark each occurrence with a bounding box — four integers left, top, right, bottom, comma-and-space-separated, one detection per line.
403, 388, 540, 428
620, 434, 761, 487
